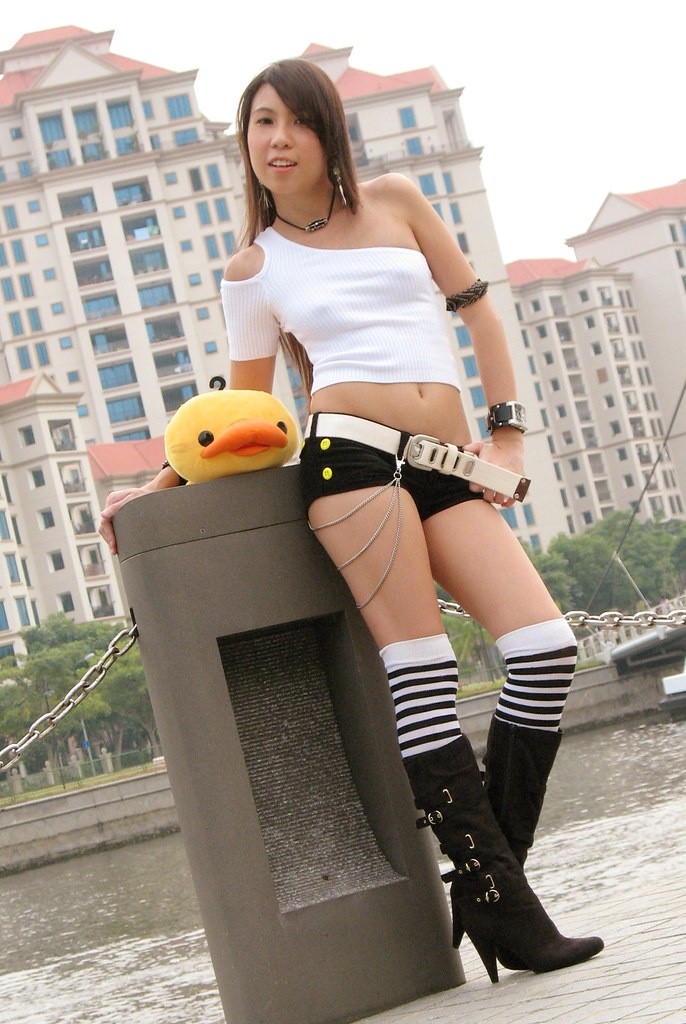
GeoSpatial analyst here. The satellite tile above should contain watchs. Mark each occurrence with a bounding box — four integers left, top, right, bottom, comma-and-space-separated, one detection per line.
486, 401, 529, 436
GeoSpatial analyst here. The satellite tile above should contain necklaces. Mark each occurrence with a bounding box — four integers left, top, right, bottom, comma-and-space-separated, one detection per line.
274, 181, 336, 232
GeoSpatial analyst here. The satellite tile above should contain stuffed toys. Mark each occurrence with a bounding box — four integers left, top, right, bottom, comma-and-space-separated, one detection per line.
165, 390, 300, 486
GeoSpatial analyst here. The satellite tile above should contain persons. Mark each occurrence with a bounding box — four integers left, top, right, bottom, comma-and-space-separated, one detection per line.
96, 62, 606, 984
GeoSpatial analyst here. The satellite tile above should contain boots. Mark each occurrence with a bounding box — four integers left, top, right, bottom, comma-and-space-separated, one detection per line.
400, 734, 606, 980
450, 715, 563, 972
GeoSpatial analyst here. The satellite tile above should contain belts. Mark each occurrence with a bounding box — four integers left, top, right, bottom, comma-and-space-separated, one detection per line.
303, 409, 531, 503
